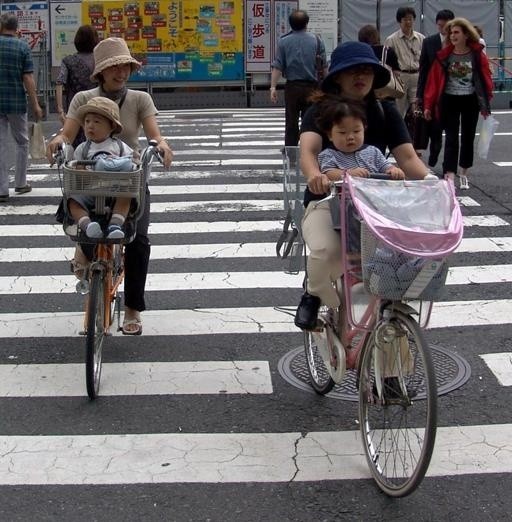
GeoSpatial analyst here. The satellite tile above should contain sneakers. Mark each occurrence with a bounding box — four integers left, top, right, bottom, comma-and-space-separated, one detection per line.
0, 194, 9, 202
107, 225, 124, 238
460, 176, 469, 189
16, 184, 31, 193
295, 291, 320, 331
85, 222, 104, 238
373, 376, 416, 400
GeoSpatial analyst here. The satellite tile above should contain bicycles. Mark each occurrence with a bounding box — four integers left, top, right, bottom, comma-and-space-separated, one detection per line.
275, 144, 470, 499
49, 140, 170, 398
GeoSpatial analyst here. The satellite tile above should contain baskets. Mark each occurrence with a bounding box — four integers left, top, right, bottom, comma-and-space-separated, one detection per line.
359, 221, 448, 302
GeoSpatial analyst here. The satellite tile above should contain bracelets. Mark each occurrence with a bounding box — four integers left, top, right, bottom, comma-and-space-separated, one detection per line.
270, 87, 276, 91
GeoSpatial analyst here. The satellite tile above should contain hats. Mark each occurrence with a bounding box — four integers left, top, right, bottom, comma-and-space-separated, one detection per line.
323, 43, 391, 89
91, 38, 141, 82
76, 97, 123, 134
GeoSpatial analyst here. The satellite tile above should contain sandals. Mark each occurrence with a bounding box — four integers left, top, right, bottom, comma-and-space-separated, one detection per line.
73, 243, 88, 280
122, 307, 143, 333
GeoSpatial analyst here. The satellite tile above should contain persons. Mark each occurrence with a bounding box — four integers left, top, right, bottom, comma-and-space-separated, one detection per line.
56, 24, 100, 147
358, 23, 402, 77
415, 9, 495, 192
307, 88, 406, 252
269, 9, 330, 167
66, 97, 139, 240
383, 6, 426, 121
293, 41, 433, 400
0, 12, 43, 204
45, 37, 173, 335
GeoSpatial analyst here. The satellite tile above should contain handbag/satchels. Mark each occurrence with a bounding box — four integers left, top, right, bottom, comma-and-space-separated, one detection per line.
378, 61, 404, 99
314, 56, 322, 78
404, 103, 429, 150
29, 122, 46, 159
